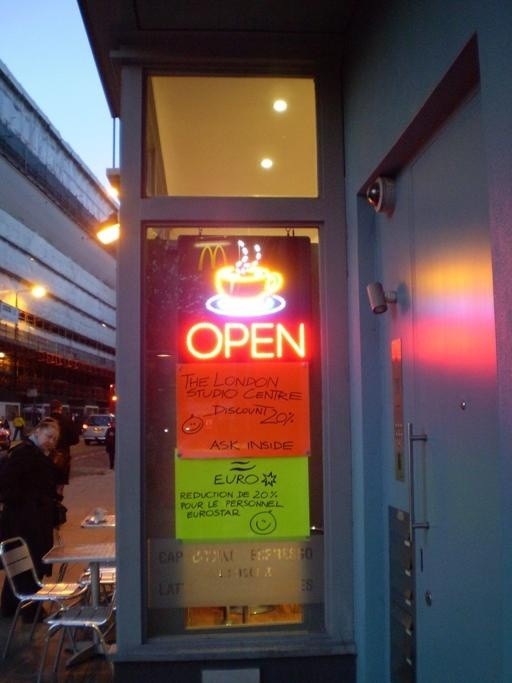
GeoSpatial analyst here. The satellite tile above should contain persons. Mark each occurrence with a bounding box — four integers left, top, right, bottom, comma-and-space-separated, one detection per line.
104, 420, 115, 469
0, 399, 80, 624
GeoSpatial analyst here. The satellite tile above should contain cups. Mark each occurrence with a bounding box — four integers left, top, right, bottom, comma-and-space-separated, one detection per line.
93, 507, 107, 523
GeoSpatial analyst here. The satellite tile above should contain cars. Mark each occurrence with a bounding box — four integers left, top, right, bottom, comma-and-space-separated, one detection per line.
82, 413, 115, 445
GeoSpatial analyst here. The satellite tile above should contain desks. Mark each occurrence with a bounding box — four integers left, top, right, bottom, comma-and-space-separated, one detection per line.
41, 542, 116, 654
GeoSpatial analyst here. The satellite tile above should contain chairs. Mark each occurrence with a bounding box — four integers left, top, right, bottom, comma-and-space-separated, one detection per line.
0, 537, 89, 660
35, 581, 117, 681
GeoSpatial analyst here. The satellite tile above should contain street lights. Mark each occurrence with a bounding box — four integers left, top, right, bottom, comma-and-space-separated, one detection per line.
15, 288, 47, 402
